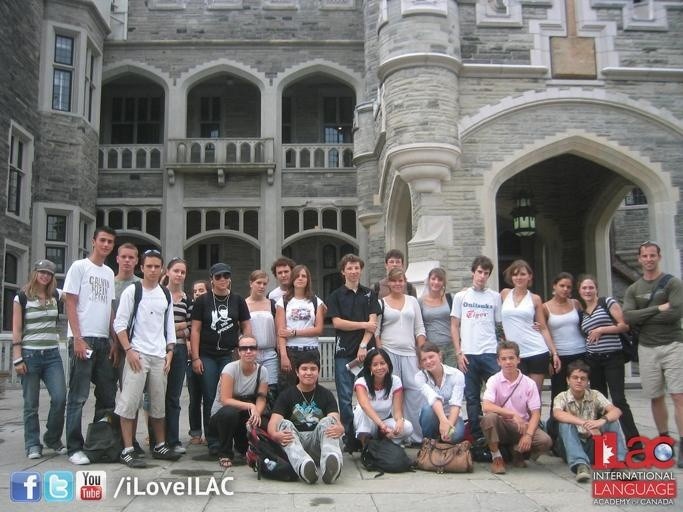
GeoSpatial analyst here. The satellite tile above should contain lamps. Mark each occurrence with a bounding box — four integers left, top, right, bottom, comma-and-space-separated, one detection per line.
510, 189, 538, 236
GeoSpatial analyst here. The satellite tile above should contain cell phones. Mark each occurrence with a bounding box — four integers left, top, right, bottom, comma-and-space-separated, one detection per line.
448, 427, 455, 437
86, 349, 94, 359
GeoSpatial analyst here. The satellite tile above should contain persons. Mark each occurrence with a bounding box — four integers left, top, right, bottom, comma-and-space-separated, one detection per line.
11, 226, 683, 486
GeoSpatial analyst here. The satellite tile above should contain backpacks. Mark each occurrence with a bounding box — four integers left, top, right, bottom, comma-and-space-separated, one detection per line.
82, 409, 124, 463
361, 437, 411, 473
245, 426, 298, 482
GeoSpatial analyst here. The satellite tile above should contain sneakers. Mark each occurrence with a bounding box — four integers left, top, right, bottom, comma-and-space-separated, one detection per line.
509, 452, 526, 467
677, 447, 683, 467
574, 463, 590, 482
491, 456, 506, 473
298, 458, 318, 484
610, 463, 635, 480
321, 452, 341, 484
28, 440, 90, 465
633, 447, 645, 460
119, 434, 201, 468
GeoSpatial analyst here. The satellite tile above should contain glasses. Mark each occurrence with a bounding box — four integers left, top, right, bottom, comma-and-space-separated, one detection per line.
144, 250, 160, 254
210, 273, 231, 280
236, 346, 257, 350
570, 376, 589, 381
170, 255, 187, 262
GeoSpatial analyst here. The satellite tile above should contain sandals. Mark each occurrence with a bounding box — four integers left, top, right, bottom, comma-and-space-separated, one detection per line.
218, 457, 232, 467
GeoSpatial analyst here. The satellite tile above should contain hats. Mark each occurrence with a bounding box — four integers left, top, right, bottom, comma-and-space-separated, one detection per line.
33, 260, 56, 276
208, 263, 232, 275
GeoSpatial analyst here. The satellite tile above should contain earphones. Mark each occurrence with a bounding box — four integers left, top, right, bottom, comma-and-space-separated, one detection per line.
210, 280, 213, 284
229, 279, 232, 283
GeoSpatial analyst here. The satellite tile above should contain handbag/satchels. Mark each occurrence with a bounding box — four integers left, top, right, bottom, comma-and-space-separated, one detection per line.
414, 437, 471, 473
469, 437, 511, 462
618, 330, 638, 362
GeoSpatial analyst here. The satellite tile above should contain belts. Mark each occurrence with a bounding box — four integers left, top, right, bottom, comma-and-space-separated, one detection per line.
283, 345, 318, 351
